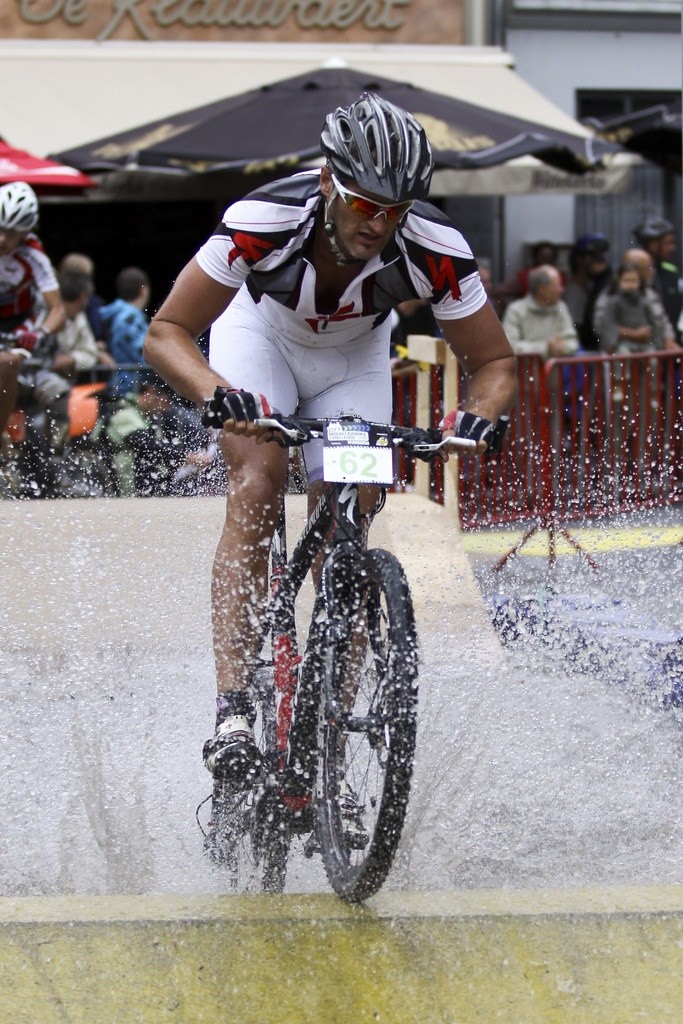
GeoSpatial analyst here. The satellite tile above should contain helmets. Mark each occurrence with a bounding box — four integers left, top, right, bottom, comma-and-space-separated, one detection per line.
569, 234, 610, 263
320, 92, 435, 203
0, 181, 39, 232
633, 215, 675, 241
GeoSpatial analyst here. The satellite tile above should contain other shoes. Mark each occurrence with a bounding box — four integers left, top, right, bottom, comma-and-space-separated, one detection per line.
201, 705, 263, 781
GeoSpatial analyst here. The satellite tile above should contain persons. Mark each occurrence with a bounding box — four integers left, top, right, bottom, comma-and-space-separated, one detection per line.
390, 215, 683, 486
143, 91, 519, 849
0, 182, 233, 499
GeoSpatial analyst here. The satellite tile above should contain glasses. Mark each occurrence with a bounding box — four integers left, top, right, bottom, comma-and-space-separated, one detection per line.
328, 167, 415, 223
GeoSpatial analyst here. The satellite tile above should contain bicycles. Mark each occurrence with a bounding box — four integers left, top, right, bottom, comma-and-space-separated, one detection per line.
195, 401, 512, 909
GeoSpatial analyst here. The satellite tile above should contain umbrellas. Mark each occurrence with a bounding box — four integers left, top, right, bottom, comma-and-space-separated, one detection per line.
1, 58, 683, 197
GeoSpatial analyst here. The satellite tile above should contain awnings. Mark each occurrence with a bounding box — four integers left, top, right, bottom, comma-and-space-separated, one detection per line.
0, 38, 632, 200
